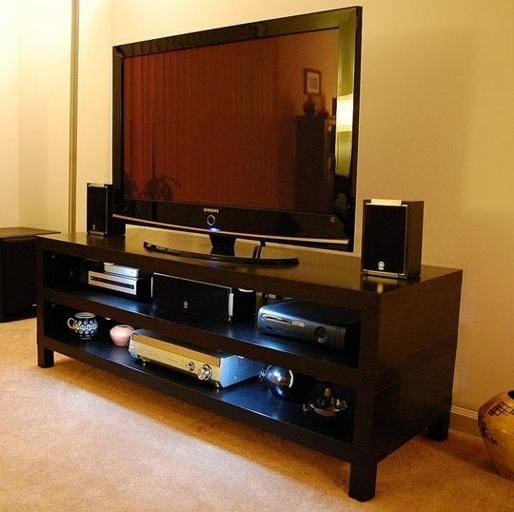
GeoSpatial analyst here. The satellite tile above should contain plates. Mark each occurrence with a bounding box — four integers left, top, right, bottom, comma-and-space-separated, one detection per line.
296, 115, 336, 211
0, 226, 61, 324
36, 227, 463, 502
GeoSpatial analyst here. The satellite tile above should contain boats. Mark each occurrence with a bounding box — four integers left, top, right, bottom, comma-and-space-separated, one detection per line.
85, 181, 125, 240
360, 198, 426, 279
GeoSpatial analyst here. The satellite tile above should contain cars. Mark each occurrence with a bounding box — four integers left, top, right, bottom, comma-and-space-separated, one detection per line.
65, 313, 98, 339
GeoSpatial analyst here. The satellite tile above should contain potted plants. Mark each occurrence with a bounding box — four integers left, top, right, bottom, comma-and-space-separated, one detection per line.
304, 67, 322, 95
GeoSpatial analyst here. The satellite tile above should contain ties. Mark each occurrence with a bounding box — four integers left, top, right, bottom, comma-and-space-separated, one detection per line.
110, 5, 365, 264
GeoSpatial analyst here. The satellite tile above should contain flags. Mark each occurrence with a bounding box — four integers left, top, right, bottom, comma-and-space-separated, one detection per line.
303, 94, 316, 115
